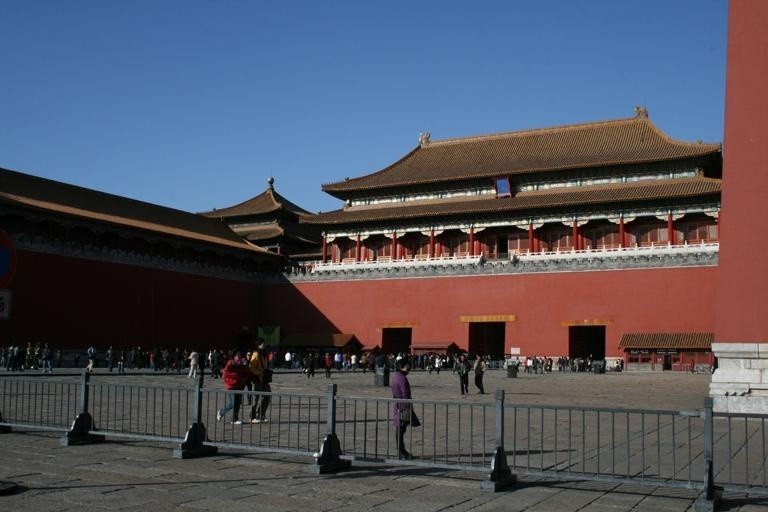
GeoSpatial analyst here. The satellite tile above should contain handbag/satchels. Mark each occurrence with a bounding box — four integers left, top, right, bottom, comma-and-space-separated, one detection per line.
263, 368, 273, 382
399, 407, 422, 427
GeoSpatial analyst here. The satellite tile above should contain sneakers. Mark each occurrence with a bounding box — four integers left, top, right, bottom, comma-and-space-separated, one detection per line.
230, 420, 243, 425
217, 409, 224, 421
248, 416, 270, 423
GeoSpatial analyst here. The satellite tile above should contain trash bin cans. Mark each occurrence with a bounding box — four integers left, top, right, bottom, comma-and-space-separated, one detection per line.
507, 365, 517, 378
375, 356, 390, 386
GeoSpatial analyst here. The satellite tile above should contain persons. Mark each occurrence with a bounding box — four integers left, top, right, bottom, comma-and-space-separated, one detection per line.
390, 358, 414, 460
246, 337, 272, 424
456, 354, 471, 395
216, 349, 248, 425
234, 351, 253, 405
515, 354, 592, 375
473, 354, 486, 395
104, 345, 115, 372
116, 349, 126, 374
1, 339, 54, 374
85, 344, 98, 373
